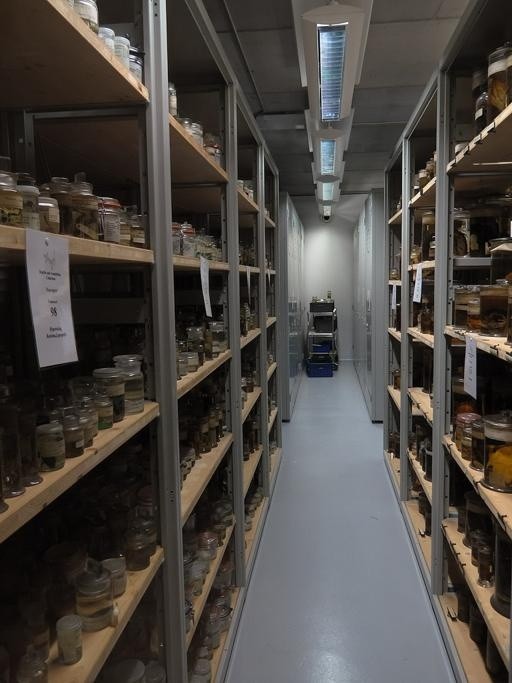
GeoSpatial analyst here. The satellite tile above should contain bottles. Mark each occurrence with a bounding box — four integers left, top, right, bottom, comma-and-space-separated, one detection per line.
1, 0, 277, 683
328, 289, 332, 302
386, 42, 512, 683
312, 296, 317, 303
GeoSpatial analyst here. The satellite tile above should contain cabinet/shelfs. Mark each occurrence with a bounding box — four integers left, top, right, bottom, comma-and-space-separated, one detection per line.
382, 0, 512, 683
0, 0, 283, 683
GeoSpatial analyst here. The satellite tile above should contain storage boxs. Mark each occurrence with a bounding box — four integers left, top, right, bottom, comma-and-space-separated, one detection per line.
304, 302, 338, 377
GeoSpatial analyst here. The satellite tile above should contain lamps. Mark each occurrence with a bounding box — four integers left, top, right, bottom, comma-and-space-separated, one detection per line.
300, 5, 366, 217
